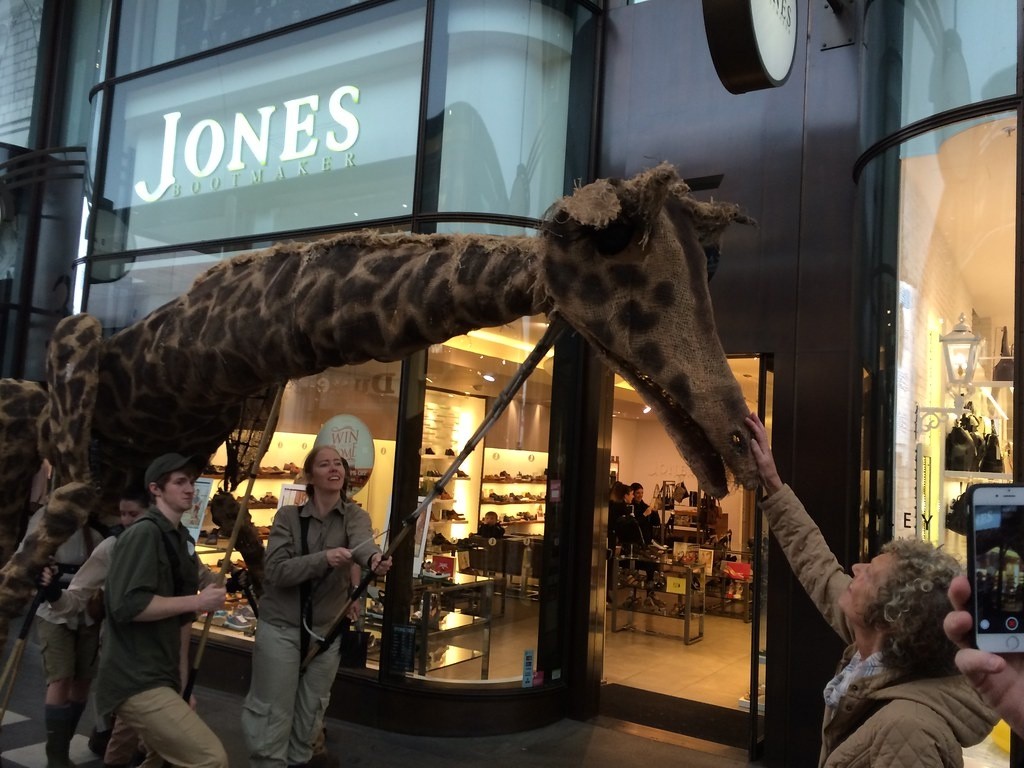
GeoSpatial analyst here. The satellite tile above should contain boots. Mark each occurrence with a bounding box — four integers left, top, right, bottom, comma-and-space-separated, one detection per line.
44, 697, 87, 768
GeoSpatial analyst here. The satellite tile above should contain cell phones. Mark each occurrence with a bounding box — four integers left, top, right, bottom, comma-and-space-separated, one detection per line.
964, 482, 1024, 655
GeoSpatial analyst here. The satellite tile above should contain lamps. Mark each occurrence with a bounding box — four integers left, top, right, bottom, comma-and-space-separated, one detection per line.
915, 311, 987, 443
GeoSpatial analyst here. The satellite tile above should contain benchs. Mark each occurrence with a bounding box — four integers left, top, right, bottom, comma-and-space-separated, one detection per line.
469, 537, 544, 601
460, 571, 507, 618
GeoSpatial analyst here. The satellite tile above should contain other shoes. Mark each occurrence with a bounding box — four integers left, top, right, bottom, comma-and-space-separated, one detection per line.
419, 447, 468, 545
198, 461, 303, 601
485, 470, 546, 522
727, 583, 743, 599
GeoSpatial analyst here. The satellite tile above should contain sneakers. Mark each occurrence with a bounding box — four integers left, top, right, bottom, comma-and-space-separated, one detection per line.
233, 607, 256, 619
411, 591, 441, 623
225, 613, 252, 628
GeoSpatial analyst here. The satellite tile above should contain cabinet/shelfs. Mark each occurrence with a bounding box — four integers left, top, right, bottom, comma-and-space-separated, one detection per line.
613, 554, 703, 646
195, 467, 296, 547
680, 545, 756, 625
415, 449, 478, 552
482, 476, 550, 524
659, 479, 712, 550
362, 572, 494, 678
938, 380, 1013, 558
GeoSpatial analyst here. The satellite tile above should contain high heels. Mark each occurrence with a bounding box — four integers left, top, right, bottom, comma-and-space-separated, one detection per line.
624, 570, 701, 617
697, 527, 733, 550
721, 564, 744, 580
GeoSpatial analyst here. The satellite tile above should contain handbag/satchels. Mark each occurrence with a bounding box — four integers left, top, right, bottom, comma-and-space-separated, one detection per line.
700, 493, 722, 525
944, 399, 1005, 473
650, 484, 675, 511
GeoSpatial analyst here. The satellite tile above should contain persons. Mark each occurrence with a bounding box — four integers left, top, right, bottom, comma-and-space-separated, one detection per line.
477, 511, 505, 539
746, 413, 1024, 768
240, 445, 392, 768
606, 481, 660, 601
0, 452, 248, 768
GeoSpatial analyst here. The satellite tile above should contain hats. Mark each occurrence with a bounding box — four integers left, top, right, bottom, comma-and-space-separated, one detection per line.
144, 453, 208, 490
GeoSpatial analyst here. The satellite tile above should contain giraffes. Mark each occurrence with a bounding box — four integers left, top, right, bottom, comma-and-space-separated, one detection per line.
1, 161, 763, 648
